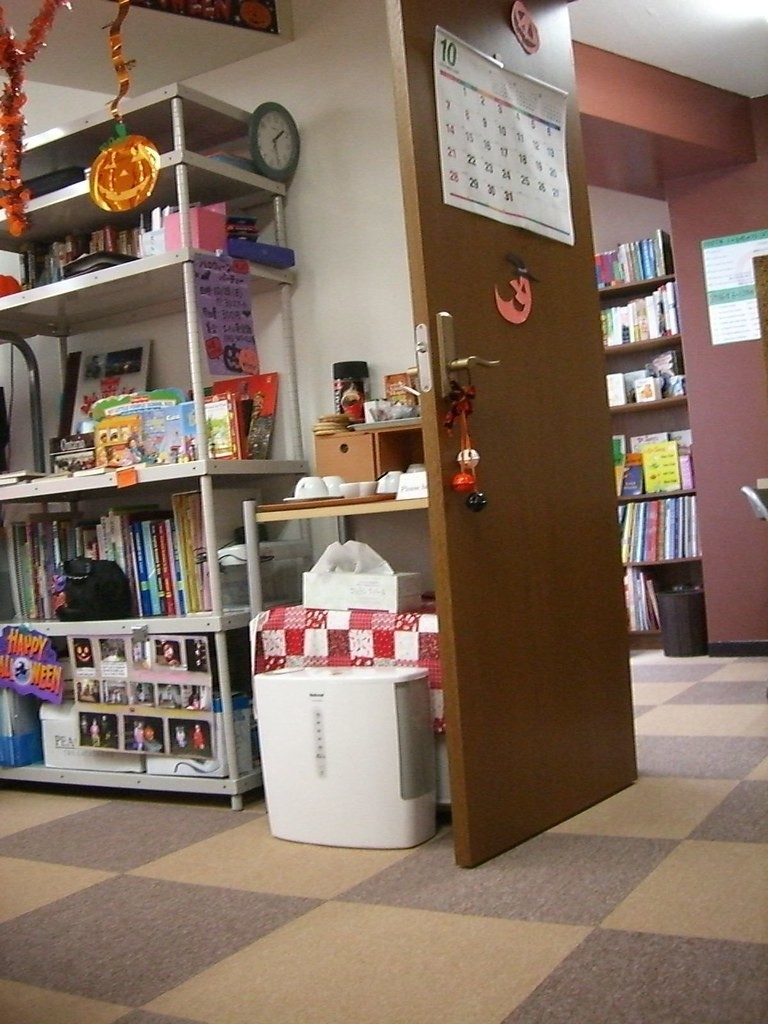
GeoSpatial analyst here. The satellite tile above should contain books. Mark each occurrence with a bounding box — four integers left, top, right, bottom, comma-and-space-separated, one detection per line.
0, 462, 277, 620
599, 281, 679, 346
613, 430, 702, 496
618, 496, 702, 563
622, 567, 668, 631
593, 229, 673, 290
19, 202, 257, 291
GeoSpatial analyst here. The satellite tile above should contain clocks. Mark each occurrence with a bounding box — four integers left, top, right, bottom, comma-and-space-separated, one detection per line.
248, 101, 302, 180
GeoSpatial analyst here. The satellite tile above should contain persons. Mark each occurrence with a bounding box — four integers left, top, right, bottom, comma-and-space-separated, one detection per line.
101, 716, 109, 738
134, 721, 144, 750
193, 724, 204, 751
145, 725, 155, 743
175, 727, 186, 747
90, 718, 100, 746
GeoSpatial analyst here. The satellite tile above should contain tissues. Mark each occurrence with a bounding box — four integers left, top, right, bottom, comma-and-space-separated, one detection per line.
302, 540, 422, 612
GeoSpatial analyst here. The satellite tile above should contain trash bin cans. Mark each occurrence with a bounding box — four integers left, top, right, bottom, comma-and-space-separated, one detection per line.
654, 587, 708, 659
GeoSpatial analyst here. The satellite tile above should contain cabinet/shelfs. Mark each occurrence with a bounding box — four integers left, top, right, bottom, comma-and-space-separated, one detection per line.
0, 83, 318, 811
240, 496, 452, 812
597, 273, 701, 650
313, 424, 426, 482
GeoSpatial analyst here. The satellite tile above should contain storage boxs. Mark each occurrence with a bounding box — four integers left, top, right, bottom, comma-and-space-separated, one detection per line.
217, 540, 308, 613
163, 203, 295, 270
39, 686, 254, 778
